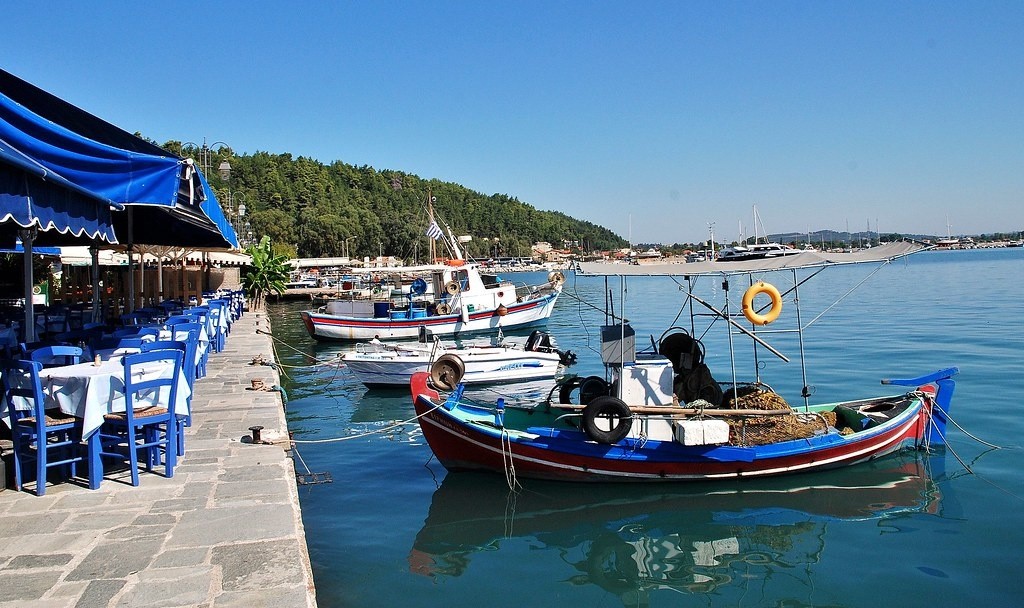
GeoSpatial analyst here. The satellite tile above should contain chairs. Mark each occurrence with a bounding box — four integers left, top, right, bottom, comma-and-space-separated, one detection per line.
0, 291, 244, 496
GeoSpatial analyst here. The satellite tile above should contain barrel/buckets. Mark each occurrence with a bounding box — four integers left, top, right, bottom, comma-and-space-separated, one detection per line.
387, 308, 408, 319
408, 308, 427, 318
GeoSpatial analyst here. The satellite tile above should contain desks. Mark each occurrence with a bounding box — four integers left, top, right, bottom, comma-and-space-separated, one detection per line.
188, 312, 228, 334
0, 360, 191, 443
193, 315, 216, 338
0, 323, 40, 360
36, 315, 71, 334
141, 328, 209, 366
93, 347, 175, 364
87, 305, 124, 318
56, 309, 92, 330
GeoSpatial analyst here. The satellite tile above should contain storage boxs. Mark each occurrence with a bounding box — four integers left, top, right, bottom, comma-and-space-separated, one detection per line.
676, 417, 730, 446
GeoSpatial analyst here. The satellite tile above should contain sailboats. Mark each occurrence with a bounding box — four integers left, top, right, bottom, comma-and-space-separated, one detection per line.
819, 217, 882, 253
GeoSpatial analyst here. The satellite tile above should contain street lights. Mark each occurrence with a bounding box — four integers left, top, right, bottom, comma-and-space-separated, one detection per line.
346, 235, 357, 258
216, 186, 246, 228
378, 242, 383, 257
180, 137, 231, 293
233, 211, 258, 251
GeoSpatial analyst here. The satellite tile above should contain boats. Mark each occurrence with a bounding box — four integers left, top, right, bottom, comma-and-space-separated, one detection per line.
408, 237, 956, 484
715, 203, 799, 261
409, 446, 947, 607
638, 248, 661, 259
300, 262, 564, 343
954, 239, 1023, 249
338, 323, 575, 388
270, 184, 469, 302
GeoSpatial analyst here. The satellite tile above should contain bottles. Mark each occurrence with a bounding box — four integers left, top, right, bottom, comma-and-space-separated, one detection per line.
77, 341, 86, 350
95, 353, 101, 366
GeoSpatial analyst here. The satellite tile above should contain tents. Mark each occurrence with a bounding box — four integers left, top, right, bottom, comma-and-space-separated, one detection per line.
0, 68, 238, 342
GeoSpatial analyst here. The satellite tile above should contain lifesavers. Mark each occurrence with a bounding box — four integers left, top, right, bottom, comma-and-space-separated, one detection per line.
559, 374, 588, 414
435, 303, 452, 316
446, 281, 460, 295
741, 281, 783, 325
582, 394, 633, 446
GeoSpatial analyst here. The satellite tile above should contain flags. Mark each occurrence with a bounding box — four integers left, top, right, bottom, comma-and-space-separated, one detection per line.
425, 222, 442, 241
